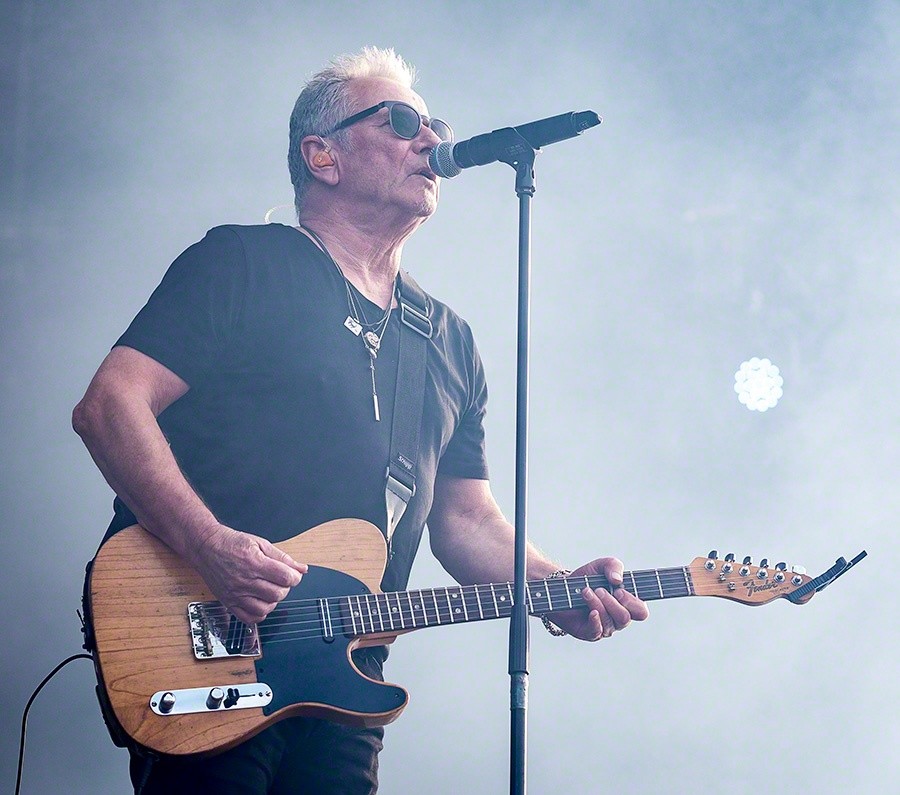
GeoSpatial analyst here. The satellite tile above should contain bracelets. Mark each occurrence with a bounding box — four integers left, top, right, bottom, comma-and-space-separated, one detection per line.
540, 570, 575, 636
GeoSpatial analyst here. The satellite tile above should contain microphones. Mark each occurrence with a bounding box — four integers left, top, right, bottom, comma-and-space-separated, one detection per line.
430, 109, 603, 179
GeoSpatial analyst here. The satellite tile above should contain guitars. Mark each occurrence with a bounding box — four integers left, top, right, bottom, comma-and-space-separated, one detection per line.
87, 517, 817, 763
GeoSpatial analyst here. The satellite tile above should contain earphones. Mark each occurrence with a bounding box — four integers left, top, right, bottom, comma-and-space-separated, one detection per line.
312, 150, 335, 169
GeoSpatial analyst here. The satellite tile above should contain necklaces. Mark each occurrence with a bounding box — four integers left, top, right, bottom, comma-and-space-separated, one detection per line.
300, 224, 396, 422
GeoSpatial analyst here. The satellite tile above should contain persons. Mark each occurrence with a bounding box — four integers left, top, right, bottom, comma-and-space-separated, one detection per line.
72, 46, 649, 794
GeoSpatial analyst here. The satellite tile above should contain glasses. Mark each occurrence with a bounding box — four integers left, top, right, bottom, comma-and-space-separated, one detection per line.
321, 100, 454, 142
